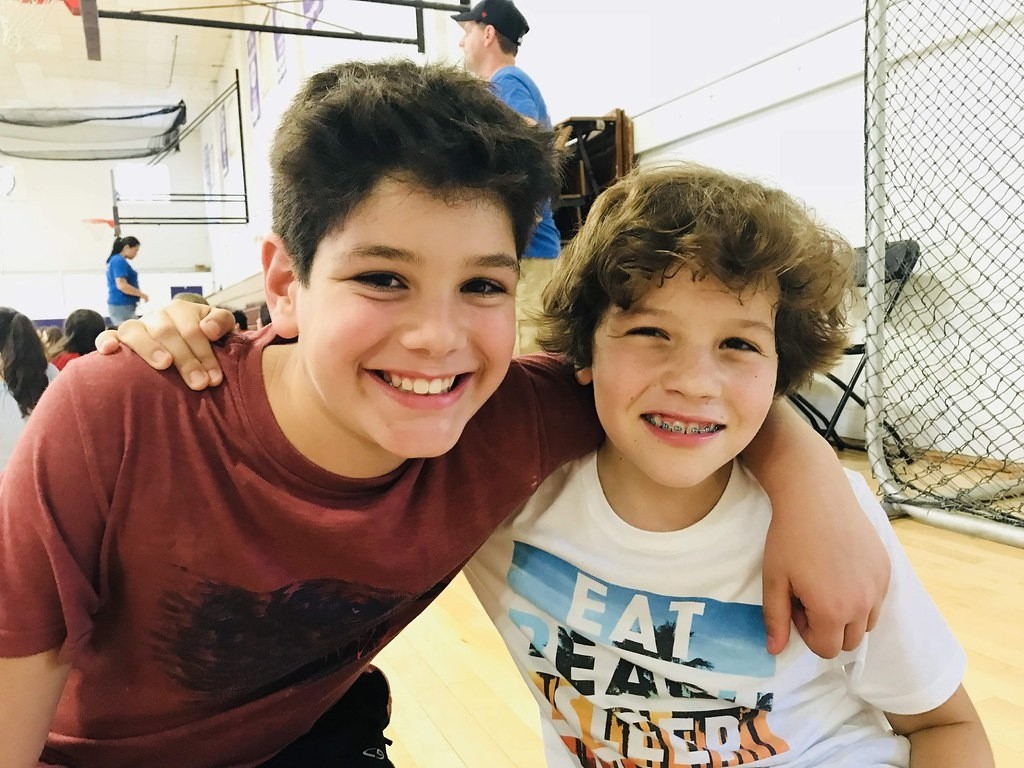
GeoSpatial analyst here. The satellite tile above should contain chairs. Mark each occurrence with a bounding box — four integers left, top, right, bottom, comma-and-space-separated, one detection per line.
783, 238, 920, 465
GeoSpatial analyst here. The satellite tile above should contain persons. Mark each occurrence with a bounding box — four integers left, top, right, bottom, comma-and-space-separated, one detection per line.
106, 236, 148, 327
93, 164, 995, 768
232, 310, 248, 333
0, 306, 117, 480
0, 60, 896, 768
256, 302, 272, 330
174, 292, 209, 306
451, 0, 563, 357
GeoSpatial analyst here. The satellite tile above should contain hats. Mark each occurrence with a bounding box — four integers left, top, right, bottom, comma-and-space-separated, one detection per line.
450, 0, 529, 48
